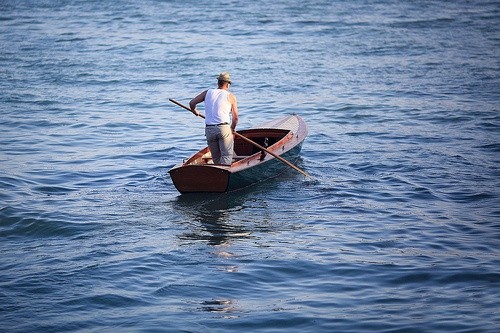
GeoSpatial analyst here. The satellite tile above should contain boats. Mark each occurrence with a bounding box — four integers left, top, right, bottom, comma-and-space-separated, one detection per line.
167, 110, 309, 195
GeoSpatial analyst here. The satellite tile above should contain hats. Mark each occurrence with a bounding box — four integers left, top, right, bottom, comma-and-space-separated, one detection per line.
217, 73, 233, 84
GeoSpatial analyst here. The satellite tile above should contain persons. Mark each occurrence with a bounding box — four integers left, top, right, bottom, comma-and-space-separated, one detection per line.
189, 72, 238, 166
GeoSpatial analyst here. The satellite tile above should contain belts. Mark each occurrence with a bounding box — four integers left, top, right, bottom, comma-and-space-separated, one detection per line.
205, 122, 230, 126
